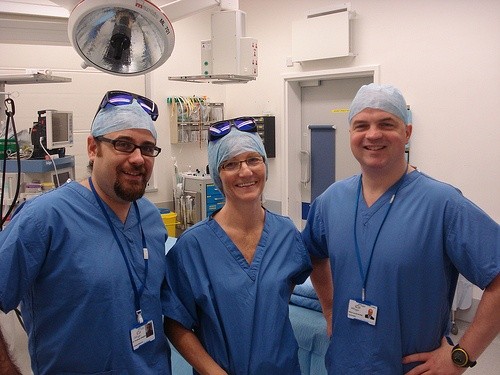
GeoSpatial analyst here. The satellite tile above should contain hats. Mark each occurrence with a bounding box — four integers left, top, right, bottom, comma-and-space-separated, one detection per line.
348, 82, 409, 128
91, 98, 157, 144
208, 127, 268, 197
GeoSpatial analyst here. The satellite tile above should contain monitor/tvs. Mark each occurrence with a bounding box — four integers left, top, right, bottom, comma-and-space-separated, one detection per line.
46, 110, 74, 150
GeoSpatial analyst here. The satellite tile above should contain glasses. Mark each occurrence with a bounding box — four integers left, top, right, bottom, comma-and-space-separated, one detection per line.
93, 90, 160, 123
218, 156, 264, 172
205, 116, 259, 142
96, 136, 162, 158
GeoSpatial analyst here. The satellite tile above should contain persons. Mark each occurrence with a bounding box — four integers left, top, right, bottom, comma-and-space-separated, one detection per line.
365, 308, 374, 320
160, 115, 312, 375
146, 323, 153, 338
302, 82, 500, 375
0, 90, 172, 375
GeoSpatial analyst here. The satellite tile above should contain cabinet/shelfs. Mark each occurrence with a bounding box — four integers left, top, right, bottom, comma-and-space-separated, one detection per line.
0, 155, 75, 232
184, 176, 226, 221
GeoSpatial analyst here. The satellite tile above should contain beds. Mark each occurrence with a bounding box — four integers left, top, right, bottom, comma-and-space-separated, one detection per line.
162, 236, 330, 375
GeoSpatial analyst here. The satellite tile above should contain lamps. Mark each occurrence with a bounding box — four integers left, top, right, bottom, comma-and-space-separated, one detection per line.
51, 0, 175, 77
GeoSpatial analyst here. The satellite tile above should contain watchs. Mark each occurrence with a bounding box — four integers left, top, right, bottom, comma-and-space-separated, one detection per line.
451, 344, 477, 368
